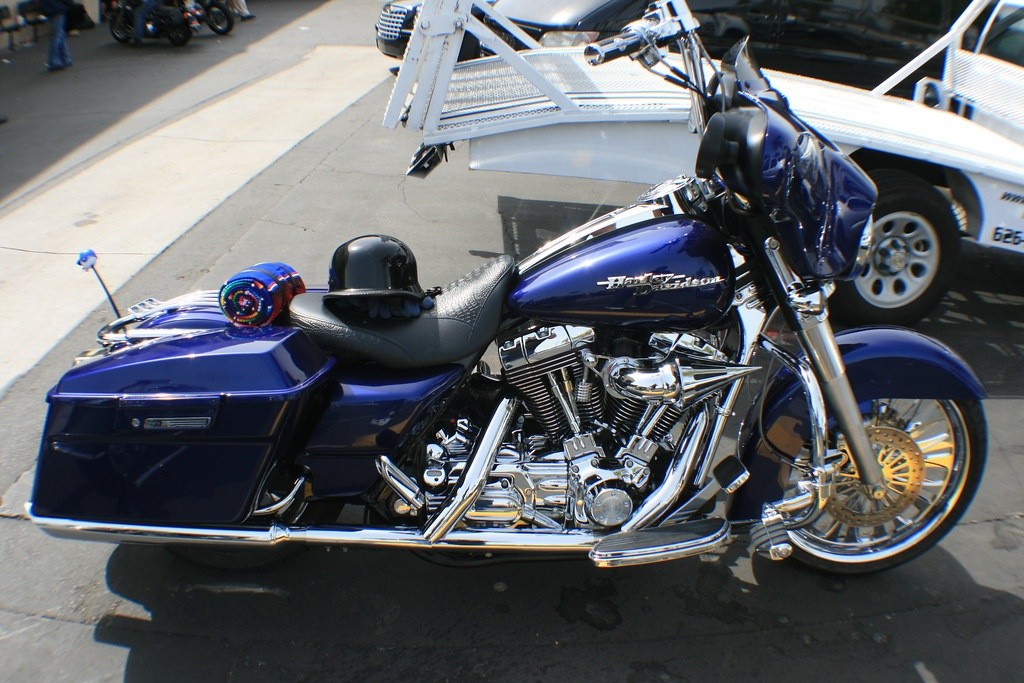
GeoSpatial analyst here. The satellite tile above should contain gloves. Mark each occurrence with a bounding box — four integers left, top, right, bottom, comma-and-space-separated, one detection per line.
368, 287, 440, 329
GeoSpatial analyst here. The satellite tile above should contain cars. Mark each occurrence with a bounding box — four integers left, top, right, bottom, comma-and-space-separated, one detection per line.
375, 0, 1024, 100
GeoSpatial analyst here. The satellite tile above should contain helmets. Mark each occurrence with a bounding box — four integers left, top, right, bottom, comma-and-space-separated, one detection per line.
323, 234, 425, 300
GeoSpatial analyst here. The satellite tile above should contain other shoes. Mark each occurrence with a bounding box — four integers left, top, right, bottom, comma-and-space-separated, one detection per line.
126, 38, 143, 48
45, 62, 73, 71
241, 14, 255, 21
0, 115, 8, 123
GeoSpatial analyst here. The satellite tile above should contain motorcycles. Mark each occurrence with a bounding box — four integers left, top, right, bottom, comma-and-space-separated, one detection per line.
106, 0, 234, 48
25, 0, 989, 579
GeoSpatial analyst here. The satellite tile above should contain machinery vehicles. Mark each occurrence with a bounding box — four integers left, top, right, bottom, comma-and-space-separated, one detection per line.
382, 0, 1024, 328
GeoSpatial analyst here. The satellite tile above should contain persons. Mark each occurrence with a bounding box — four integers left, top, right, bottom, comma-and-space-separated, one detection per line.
33, 0, 74, 70
228, 0, 257, 20
